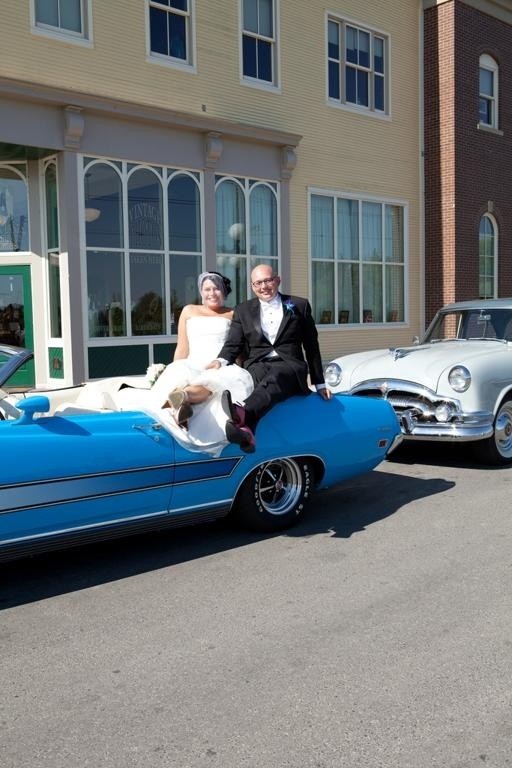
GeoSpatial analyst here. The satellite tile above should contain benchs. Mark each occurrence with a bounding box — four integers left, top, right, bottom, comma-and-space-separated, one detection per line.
57, 381, 147, 413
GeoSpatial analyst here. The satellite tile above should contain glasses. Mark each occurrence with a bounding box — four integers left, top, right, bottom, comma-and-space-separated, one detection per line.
253, 275, 276, 287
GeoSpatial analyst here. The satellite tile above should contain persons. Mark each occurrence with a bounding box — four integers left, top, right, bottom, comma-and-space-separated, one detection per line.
202, 263, 334, 453
138, 271, 254, 461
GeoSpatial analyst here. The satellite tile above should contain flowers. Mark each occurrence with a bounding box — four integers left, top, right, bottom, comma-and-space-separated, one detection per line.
145, 363, 165, 383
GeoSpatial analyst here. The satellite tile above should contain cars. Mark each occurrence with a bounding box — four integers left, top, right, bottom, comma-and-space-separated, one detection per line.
323, 297, 512, 466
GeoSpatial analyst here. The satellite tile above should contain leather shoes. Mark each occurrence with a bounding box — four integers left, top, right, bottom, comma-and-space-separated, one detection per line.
225, 422, 256, 453
221, 390, 246, 428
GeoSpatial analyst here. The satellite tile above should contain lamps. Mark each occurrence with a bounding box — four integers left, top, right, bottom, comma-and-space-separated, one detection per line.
85, 173, 100, 222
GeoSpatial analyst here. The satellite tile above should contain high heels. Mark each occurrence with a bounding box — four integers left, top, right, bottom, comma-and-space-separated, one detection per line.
168, 390, 193, 431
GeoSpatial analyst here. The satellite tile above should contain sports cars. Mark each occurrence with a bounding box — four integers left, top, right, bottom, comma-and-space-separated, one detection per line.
0, 350, 406, 563
